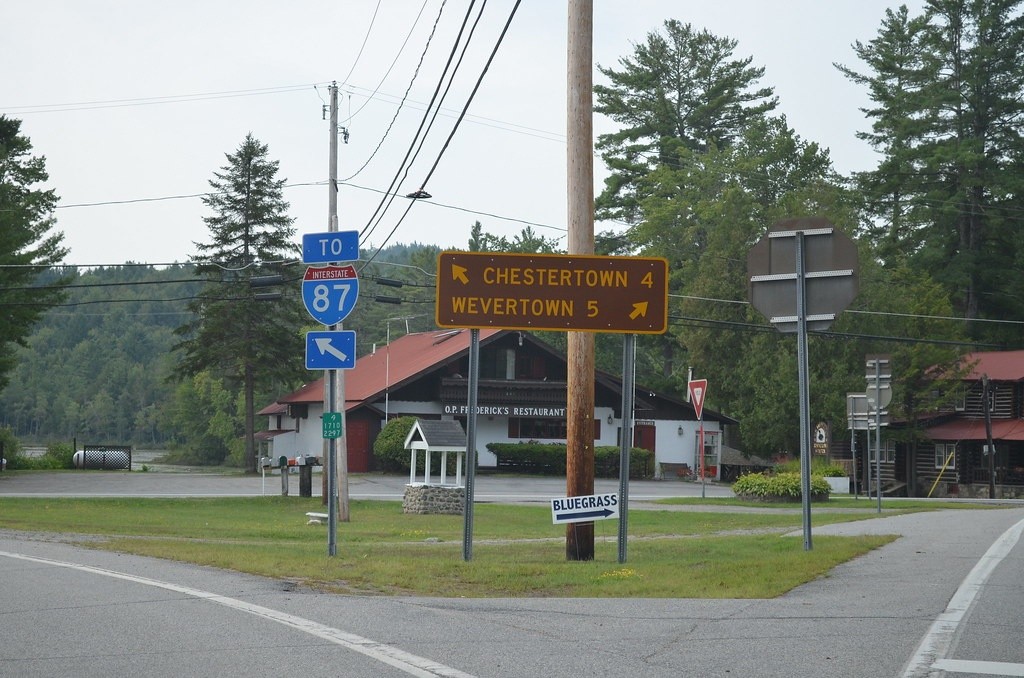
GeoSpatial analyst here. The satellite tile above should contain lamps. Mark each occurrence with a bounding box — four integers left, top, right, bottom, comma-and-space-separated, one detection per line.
608, 414, 612, 423
678, 426, 683, 435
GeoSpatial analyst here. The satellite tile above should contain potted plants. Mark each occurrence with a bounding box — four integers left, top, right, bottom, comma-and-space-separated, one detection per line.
732, 471, 832, 503
